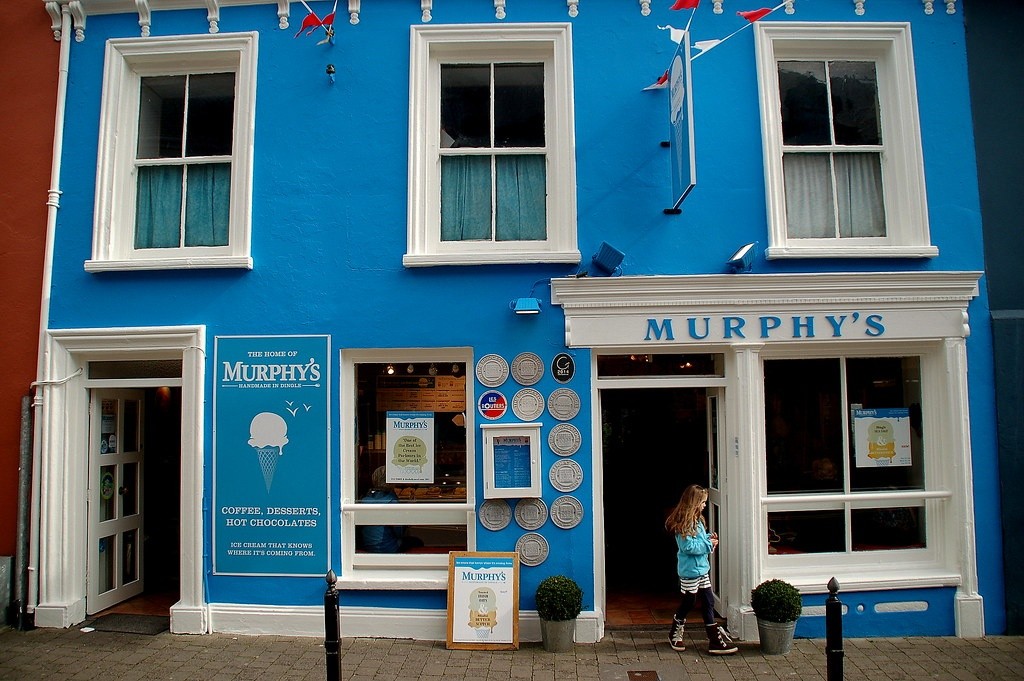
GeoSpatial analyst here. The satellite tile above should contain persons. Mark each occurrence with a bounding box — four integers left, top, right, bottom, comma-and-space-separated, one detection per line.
664, 481, 740, 655
359, 465, 424, 553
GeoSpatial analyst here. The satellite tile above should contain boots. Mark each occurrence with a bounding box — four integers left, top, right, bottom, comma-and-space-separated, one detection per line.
707, 622, 739, 654
668, 614, 686, 651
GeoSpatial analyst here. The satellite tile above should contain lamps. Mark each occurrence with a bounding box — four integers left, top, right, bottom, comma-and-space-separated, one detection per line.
510, 297, 542, 315
592, 240, 626, 276
725, 241, 760, 274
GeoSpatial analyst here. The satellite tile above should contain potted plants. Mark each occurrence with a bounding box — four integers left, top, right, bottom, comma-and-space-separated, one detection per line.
751, 578, 803, 656
535, 574, 584, 653
